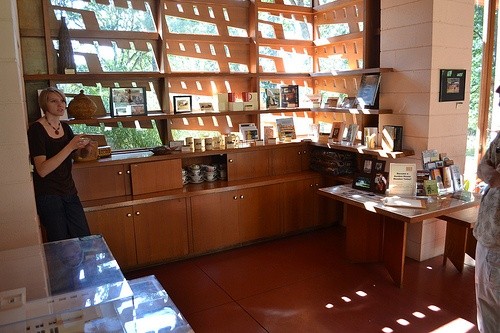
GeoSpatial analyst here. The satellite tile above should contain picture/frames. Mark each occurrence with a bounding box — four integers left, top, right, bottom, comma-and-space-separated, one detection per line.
439, 68, 467, 103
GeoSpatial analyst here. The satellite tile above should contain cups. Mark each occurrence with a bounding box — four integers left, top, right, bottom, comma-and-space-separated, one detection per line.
205, 138, 212, 150
186, 137, 193, 149
194, 139, 201, 150
228, 93, 237, 102
78, 138, 90, 160
182, 164, 226, 183
242, 92, 252, 102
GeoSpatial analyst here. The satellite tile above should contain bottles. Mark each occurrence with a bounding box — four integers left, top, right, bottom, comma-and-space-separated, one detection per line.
67, 90, 97, 119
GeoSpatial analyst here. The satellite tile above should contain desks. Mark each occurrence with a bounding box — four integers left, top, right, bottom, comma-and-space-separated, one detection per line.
315, 181, 476, 288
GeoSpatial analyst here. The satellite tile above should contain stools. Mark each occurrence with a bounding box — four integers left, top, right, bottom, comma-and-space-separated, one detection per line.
435, 206, 481, 274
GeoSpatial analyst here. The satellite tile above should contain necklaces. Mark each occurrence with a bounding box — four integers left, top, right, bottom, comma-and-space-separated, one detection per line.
46, 116, 61, 136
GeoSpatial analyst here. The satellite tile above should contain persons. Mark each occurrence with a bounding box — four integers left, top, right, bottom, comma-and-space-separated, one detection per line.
472, 85, 500, 332
28, 87, 100, 293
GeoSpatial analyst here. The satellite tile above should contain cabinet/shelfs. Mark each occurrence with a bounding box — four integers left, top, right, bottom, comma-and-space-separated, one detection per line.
16, 0, 414, 159
0, 232, 199, 333
71, 145, 353, 271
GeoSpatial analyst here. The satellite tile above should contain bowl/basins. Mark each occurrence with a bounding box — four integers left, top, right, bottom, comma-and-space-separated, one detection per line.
149, 146, 178, 154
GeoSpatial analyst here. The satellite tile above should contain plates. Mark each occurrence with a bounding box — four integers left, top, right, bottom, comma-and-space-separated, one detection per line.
217, 176, 226, 180
184, 181, 188, 184
204, 178, 217, 182
189, 180, 204, 183
